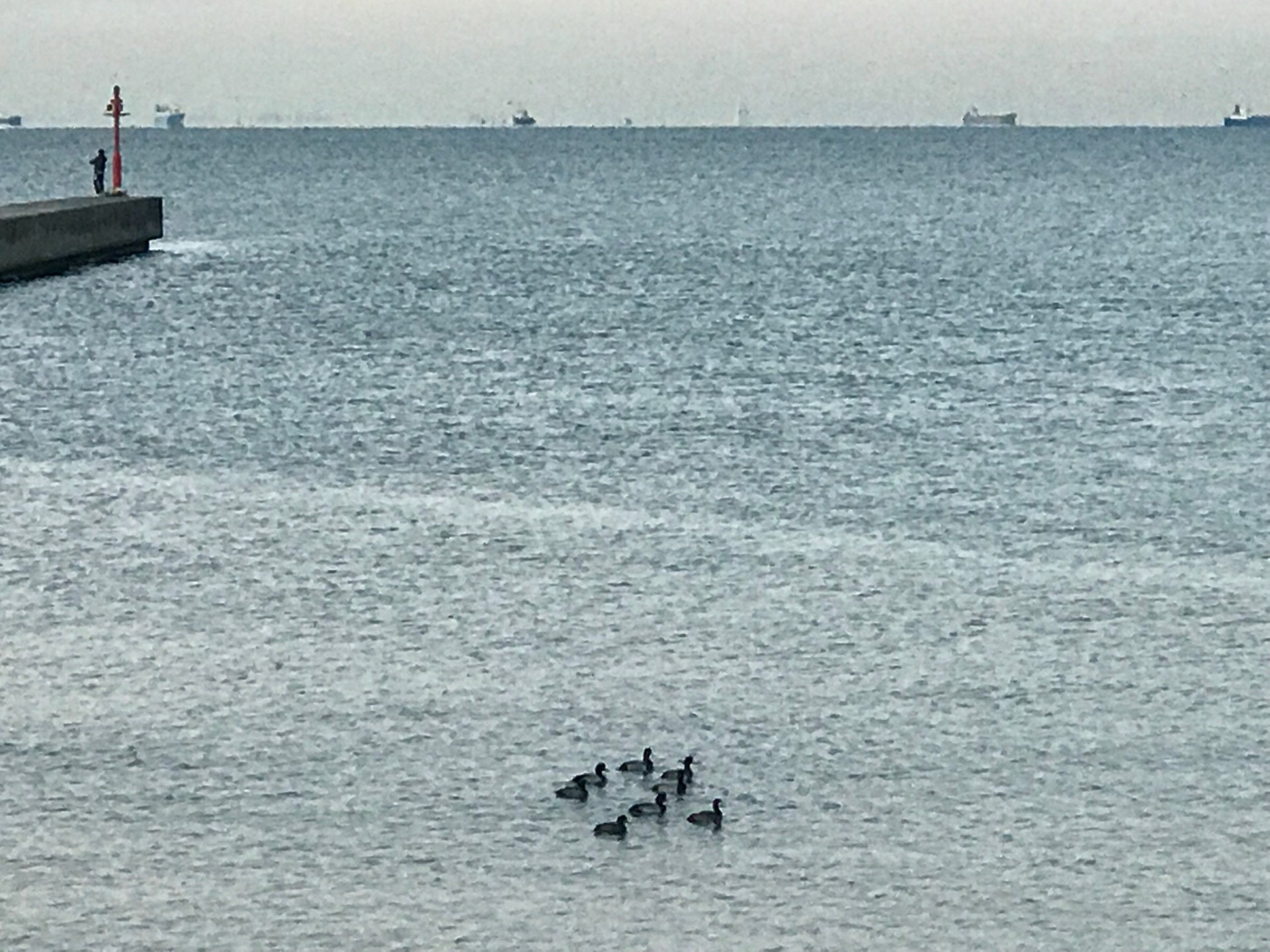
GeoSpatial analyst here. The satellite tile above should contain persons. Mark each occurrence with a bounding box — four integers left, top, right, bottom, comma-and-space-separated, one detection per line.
89, 149, 107, 197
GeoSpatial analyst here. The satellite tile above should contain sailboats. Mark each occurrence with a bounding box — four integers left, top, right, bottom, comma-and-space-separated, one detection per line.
512, 98, 537, 127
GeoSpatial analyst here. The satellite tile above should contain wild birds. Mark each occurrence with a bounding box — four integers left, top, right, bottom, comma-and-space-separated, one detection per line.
593, 815, 631, 840
686, 798, 722, 829
617, 746, 693, 796
572, 762, 610, 787
629, 791, 667, 818
555, 780, 588, 802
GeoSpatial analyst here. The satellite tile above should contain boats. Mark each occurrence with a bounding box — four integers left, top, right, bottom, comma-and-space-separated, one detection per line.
961, 104, 1019, 127
1224, 103, 1270, 127
153, 103, 185, 130
